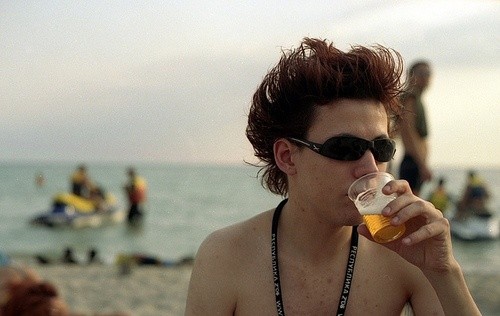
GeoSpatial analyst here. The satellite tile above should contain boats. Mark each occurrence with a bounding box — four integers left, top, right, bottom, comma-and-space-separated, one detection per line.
49, 193, 123, 229
450, 217, 500, 242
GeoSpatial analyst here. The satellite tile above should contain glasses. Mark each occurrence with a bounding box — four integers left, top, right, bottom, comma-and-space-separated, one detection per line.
273, 134, 396, 162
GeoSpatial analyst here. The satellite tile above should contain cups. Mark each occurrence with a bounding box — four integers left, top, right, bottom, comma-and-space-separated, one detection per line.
347, 172, 406, 244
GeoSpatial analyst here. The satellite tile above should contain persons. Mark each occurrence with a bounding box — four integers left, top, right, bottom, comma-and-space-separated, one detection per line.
184, 34, 483, 316
427, 168, 492, 224
396, 60, 433, 195
0, 161, 160, 316
